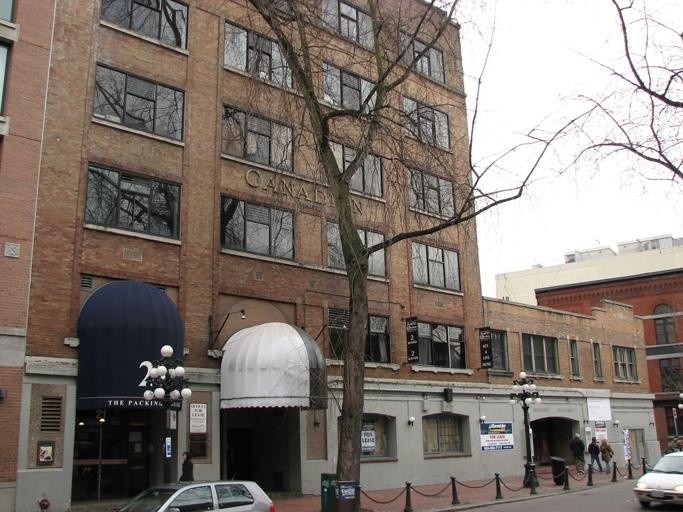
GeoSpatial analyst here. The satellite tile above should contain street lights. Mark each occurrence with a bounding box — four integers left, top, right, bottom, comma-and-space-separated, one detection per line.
143, 345, 190, 483
508, 372, 542, 486
677, 392, 683, 409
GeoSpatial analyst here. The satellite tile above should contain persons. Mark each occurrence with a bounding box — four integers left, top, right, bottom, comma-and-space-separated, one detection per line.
588, 437, 602, 473
176, 451, 193, 481
671, 435, 680, 452
598, 438, 615, 475
569, 432, 585, 476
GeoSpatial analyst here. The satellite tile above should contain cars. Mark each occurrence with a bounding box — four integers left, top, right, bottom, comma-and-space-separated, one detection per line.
116, 478, 276, 512
632, 450, 683, 508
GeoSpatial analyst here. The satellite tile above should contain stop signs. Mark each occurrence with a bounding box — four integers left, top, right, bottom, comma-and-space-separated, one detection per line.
672, 408, 677, 418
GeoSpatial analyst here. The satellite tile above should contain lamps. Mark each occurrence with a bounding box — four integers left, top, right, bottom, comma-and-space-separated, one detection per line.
208, 308, 245, 350
613, 420, 619, 428
407, 416, 415, 426
394, 302, 407, 311
479, 415, 486, 424
300, 322, 346, 341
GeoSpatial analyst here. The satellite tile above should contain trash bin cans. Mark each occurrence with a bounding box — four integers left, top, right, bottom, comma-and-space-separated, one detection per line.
321, 473, 337, 512
549, 456, 566, 485
